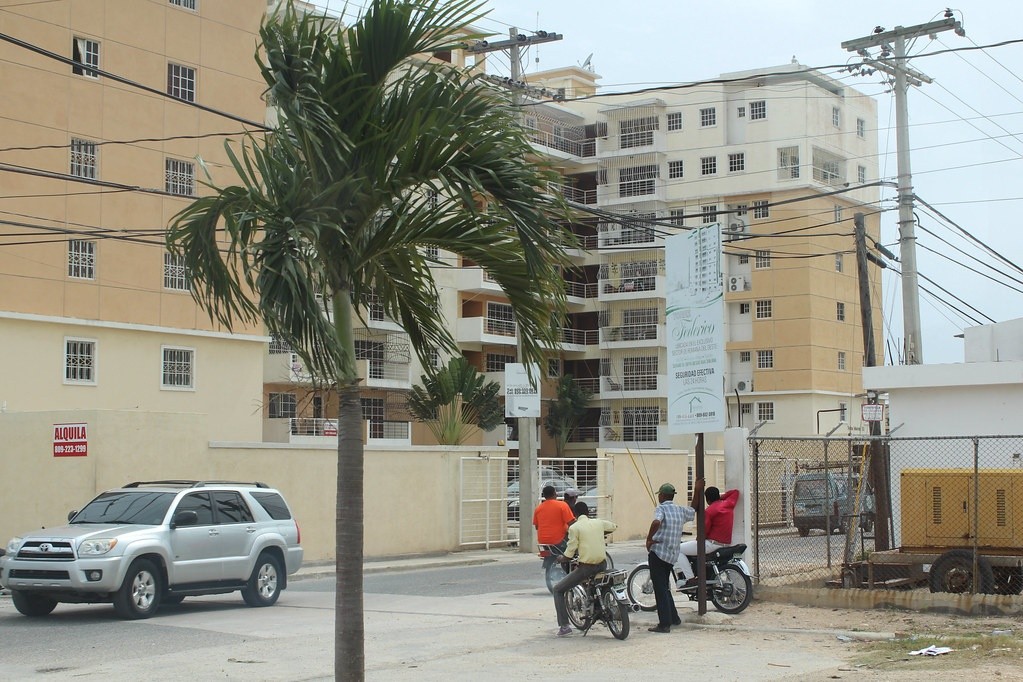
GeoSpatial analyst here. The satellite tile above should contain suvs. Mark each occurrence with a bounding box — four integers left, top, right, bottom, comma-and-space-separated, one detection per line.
0, 480, 303, 618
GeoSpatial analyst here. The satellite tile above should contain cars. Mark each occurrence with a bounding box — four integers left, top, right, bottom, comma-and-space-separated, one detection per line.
507, 466, 597, 520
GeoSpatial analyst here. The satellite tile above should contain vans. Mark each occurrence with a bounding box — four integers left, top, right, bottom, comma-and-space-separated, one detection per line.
793, 473, 875, 536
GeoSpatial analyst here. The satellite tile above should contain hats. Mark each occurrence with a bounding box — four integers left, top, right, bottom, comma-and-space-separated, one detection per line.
564, 488, 583, 497
655, 483, 677, 495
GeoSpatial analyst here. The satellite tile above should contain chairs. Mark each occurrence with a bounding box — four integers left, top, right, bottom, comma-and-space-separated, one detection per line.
606, 427, 621, 441
305, 416, 320, 436
607, 376, 624, 391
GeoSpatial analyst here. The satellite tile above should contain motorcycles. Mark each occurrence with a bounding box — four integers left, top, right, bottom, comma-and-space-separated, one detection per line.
626, 531, 759, 614
556, 555, 641, 640
537, 544, 615, 594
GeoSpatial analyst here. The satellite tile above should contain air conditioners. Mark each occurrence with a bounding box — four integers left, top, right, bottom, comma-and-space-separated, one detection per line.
729, 220, 743, 231
728, 276, 746, 292
732, 378, 751, 393
731, 235, 744, 242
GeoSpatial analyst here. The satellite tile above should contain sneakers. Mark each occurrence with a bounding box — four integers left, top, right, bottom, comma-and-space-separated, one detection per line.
557, 627, 573, 636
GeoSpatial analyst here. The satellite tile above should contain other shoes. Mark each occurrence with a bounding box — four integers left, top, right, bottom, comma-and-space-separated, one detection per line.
681, 578, 697, 587
648, 626, 670, 633
671, 620, 681, 625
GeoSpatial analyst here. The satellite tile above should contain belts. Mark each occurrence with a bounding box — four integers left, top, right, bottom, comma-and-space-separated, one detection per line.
582, 560, 605, 567
708, 540, 728, 547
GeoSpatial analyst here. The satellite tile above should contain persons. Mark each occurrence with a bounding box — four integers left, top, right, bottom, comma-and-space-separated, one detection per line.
646, 478, 706, 633
562, 488, 585, 526
677, 486, 741, 588
533, 486, 576, 552
553, 502, 616, 637
